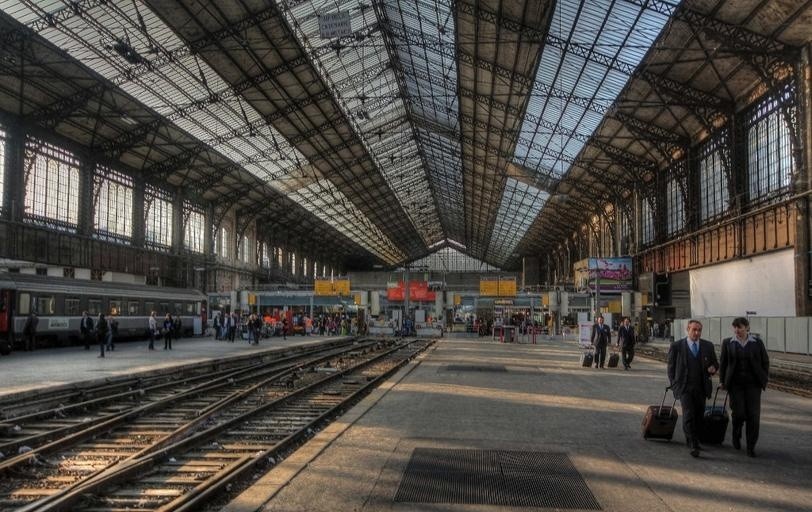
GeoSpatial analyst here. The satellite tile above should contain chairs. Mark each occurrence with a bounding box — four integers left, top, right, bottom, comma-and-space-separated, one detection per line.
734, 437, 758, 458
686, 438, 705, 458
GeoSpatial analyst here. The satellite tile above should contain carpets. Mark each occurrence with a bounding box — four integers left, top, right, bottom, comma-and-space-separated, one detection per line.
690, 340, 700, 357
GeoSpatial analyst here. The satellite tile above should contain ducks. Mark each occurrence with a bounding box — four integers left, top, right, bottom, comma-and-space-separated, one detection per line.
697, 404, 730, 449
639, 405, 678, 444
578, 352, 620, 369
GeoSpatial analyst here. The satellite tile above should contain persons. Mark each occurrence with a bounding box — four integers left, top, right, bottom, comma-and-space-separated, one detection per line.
588, 260, 632, 281
666, 320, 719, 458
201, 305, 413, 346
612, 318, 674, 342
80, 308, 120, 359
163, 313, 173, 350
0, 301, 13, 341
615, 316, 636, 370
147, 311, 158, 350
720, 317, 770, 459
174, 314, 183, 340
590, 316, 611, 369
23, 311, 39, 351
426, 309, 554, 339
